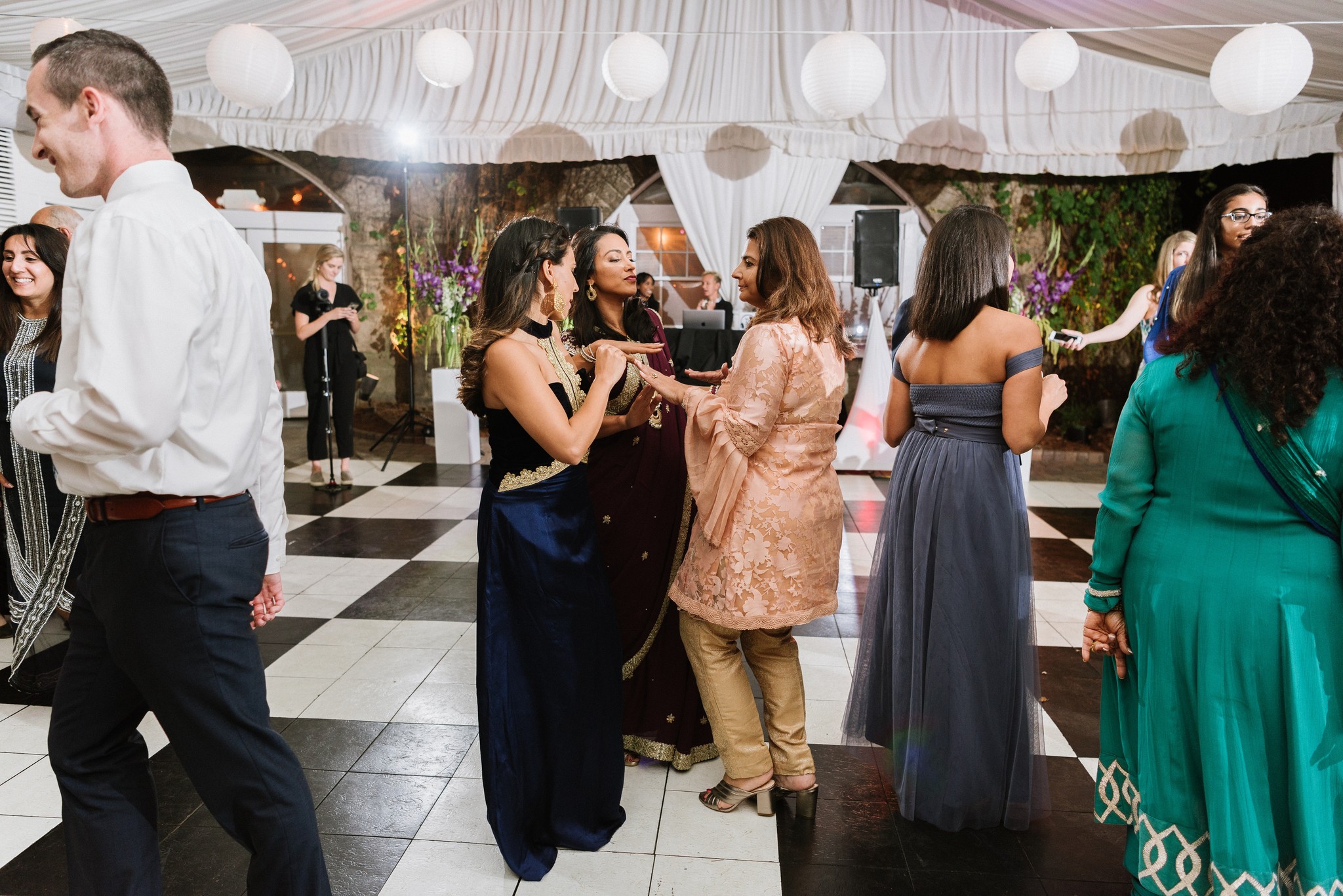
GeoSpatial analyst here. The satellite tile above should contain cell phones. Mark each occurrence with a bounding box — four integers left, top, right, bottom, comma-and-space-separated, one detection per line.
1047, 330, 1083, 346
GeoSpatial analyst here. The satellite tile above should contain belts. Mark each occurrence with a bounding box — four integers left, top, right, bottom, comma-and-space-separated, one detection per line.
86, 494, 225, 521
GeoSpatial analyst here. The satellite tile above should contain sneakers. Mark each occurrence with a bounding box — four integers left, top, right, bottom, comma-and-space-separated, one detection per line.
309, 471, 325, 486
340, 469, 354, 485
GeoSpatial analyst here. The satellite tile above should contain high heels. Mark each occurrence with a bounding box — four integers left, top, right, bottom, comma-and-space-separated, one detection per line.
780, 775, 819, 817
699, 779, 774, 817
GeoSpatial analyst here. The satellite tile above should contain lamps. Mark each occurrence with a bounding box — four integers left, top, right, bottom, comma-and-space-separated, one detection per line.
353, 373, 382, 411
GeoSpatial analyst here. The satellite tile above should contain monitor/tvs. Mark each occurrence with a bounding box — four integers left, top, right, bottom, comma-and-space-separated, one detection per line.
682, 309, 726, 330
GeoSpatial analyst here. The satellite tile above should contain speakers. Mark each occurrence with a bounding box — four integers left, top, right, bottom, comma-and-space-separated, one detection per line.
853, 208, 900, 288
556, 205, 600, 243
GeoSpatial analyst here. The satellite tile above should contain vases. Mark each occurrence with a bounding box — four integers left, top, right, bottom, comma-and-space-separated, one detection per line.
438, 319, 464, 368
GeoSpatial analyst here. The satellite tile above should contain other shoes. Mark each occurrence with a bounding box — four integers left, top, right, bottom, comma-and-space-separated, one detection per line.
622, 749, 641, 767
1, 621, 15, 637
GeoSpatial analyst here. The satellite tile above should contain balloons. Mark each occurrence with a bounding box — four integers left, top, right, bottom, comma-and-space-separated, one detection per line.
800, 33, 884, 120
28, 19, 84, 56
415, 27, 473, 88
1014, 29, 1081, 92
1210, 24, 1313, 115
206, 24, 294, 110
602, 32, 669, 102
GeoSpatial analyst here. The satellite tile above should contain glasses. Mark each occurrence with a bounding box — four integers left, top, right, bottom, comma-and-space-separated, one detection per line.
1222, 211, 1271, 222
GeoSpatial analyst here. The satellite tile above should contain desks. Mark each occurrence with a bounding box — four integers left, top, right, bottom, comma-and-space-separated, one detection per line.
430, 366, 484, 464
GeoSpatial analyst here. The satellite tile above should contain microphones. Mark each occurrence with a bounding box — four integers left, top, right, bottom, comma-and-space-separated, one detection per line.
311, 289, 329, 304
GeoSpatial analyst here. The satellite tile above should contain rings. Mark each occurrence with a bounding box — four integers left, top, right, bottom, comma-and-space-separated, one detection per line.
1090, 641, 1098, 653
652, 373, 658, 380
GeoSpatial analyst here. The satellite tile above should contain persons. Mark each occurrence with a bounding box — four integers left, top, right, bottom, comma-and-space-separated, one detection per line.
562, 224, 720, 770
634, 272, 659, 313
1, 612, 14, 638
29, 205, 84, 240
1047, 230, 1198, 379
6, 28, 335, 896
632, 216, 857, 824
696, 271, 733, 330
892, 203, 991, 355
1142, 184, 1274, 365
458, 216, 665, 881
289, 243, 368, 489
1082, 207, 1343, 896
842, 209, 1068, 830
0, 224, 79, 691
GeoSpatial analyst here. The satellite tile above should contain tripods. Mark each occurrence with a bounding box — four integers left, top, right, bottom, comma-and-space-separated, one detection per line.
371, 274, 434, 471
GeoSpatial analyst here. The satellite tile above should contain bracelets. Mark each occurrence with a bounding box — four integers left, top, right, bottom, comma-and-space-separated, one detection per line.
709, 385, 720, 395
1086, 602, 1124, 612
579, 344, 596, 363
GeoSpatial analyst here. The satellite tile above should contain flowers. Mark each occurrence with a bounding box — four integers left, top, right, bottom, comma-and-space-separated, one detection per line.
1002, 214, 1097, 366
409, 256, 477, 368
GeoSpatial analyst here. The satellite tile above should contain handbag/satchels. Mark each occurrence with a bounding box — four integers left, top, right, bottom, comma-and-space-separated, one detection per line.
351, 351, 368, 378
